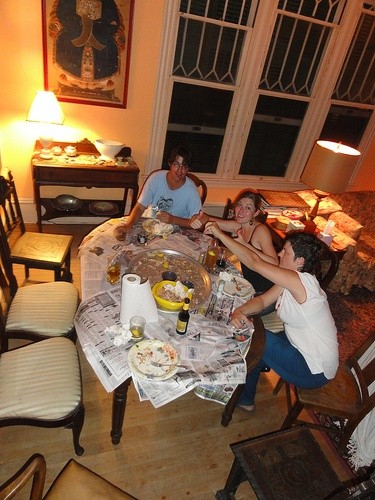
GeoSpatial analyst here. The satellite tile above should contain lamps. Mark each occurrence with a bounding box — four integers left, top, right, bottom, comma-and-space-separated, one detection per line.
300, 139, 361, 230
26, 90, 64, 160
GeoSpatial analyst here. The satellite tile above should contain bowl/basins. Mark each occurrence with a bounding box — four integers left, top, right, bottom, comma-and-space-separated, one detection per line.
281, 209, 304, 221
95, 140, 124, 162
152, 280, 193, 311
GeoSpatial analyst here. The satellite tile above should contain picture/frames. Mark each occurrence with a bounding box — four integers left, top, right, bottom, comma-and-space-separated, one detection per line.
42, 0, 135, 109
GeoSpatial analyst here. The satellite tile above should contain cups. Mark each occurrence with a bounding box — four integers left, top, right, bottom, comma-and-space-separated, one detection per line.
216, 299, 233, 324
116, 226, 127, 242
130, 316, 146, 338
162, 271, 177, 281
52, 146, 77, 156
181, 280, 194, 289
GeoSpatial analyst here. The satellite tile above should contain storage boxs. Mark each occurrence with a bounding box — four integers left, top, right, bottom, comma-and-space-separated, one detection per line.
275, 216, 292, 230
282, 209, 303, 220
328, 211, 363, 238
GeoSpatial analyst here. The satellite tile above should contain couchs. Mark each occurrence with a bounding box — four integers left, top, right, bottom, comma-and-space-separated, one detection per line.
318, 190, 375, 294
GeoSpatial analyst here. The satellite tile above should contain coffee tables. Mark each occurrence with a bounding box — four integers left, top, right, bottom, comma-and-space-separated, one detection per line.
266, 218, 345, 296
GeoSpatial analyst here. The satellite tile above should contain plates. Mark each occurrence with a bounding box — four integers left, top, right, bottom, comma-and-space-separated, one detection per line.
128, 339, 180, 382
142, 218, 174, 235
215, 276, 254, 299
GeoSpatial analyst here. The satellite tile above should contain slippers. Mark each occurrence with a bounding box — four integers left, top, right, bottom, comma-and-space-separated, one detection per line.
237, 404, 255, 413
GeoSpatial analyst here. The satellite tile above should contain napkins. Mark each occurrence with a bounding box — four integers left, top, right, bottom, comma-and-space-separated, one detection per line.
108, 323, 132, 346
164, 281, 194, 299
219, 271, 233, 282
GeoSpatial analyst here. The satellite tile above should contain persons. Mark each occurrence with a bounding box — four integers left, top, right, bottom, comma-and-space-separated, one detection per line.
204, 220, 339, 414
114, 145, 202, 235
190, 187, 280, 316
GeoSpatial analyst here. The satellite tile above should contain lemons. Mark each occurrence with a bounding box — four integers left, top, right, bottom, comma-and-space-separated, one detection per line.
132, 329, 139, 338
108, 266, 115, 271
208, 250, 215, 256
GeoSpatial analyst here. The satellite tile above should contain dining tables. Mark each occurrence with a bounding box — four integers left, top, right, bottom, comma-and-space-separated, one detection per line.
74, 216, 268, 445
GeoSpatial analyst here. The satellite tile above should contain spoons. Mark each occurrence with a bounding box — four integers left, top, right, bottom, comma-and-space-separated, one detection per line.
233, 277, 241, 291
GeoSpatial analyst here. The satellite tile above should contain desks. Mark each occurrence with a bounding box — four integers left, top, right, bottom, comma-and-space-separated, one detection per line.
31, 139, 140, 231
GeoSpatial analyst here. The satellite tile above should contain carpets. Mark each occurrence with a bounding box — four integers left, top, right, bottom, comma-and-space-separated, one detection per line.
313, 289, 375, 474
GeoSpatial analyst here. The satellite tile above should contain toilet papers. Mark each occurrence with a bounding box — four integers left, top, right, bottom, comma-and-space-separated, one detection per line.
120, 274, 159, 324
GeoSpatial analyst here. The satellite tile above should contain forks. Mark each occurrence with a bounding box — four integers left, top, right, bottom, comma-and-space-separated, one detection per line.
151, 361, 188, 368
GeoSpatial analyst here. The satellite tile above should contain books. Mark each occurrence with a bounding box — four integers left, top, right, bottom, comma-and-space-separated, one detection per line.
256, 189, 311, 215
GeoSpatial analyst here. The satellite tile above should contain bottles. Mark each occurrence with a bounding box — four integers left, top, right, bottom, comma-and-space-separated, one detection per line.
205, 280, 226, 321
205, 237, 226, 275
176, 297, 190, 335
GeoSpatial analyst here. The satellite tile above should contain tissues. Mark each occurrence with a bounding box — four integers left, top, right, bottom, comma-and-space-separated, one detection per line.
316, 220, 336, 246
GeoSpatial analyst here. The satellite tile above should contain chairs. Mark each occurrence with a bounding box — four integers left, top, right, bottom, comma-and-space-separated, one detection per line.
214, 198, 375, 500
0, 169, 207, 500
